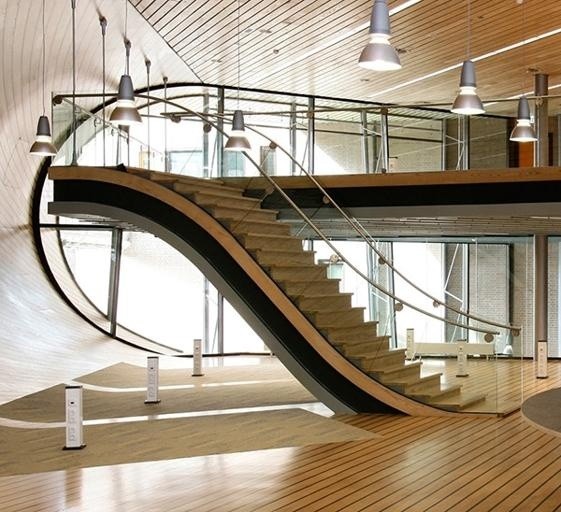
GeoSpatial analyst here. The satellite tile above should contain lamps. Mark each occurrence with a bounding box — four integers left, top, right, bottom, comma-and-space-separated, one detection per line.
109, 1, 144, 127
509, 0, 538, 143
29, 1, 57, 156
225, 1, 252, 152
357, 0, 402, 72
451, 0, 487, 117
